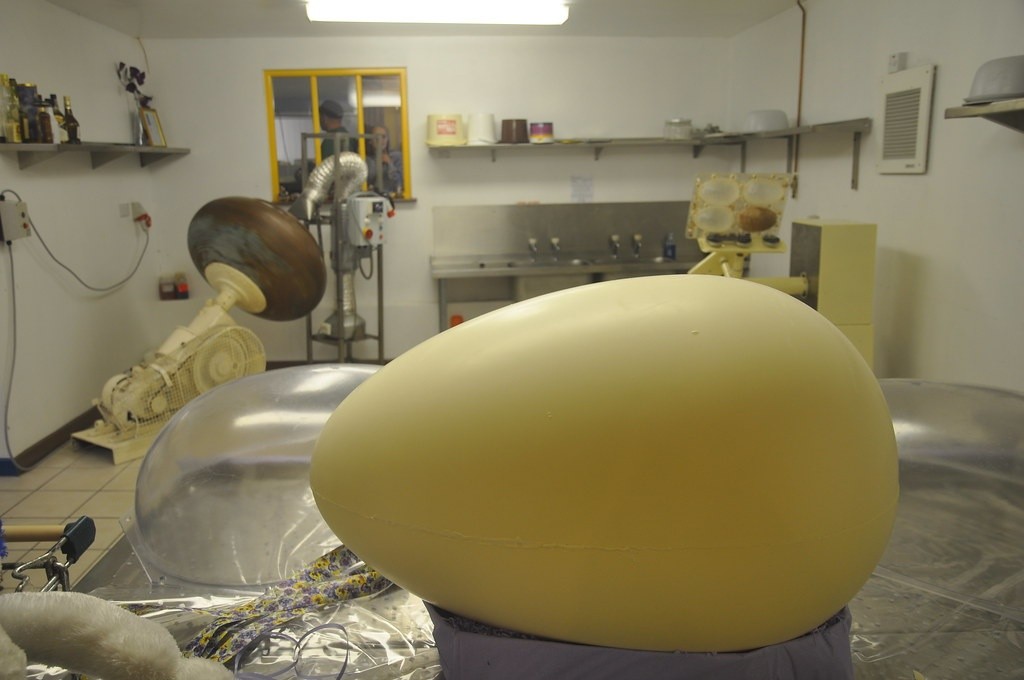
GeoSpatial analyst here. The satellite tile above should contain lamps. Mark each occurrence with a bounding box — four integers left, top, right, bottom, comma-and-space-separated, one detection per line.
306, 0, 570, 25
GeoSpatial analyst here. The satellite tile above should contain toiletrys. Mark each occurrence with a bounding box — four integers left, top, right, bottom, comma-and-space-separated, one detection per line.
663, 231, 677, 262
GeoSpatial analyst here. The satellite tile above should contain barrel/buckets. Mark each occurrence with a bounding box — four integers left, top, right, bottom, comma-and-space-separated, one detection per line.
424, 114, 466, 147
467, 115, 497, 146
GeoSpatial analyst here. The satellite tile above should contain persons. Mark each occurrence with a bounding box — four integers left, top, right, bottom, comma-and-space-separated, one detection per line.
295, 100, 358, 180
365, 125, 403, 192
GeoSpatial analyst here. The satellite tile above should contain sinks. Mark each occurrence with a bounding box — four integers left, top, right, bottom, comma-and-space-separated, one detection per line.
590, 255, 678, 282
507, 258, 595, 302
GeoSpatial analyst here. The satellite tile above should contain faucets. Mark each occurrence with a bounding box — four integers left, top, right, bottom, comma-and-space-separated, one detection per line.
550, 237, 564, 265
633, 234, 642, 259
528, 238, 540, 264
611, 234, 620, 259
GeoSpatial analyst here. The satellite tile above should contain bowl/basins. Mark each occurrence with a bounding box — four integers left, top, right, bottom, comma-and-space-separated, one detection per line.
743, 109, 789, 132
662, 119, 692, 140
529, 122, 553, 143
962, 54, 1024, 105
498, 119, 528, 142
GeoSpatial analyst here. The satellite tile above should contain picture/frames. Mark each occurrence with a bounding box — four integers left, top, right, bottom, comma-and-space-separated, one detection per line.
139, 108, 167, 146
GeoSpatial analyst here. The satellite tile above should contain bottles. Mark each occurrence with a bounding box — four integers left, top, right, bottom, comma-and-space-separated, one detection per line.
0, 74, 79, 143
664, 232, 675, 261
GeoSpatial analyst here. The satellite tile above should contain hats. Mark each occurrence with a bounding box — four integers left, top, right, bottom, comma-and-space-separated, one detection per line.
320, 100, 343, 117
